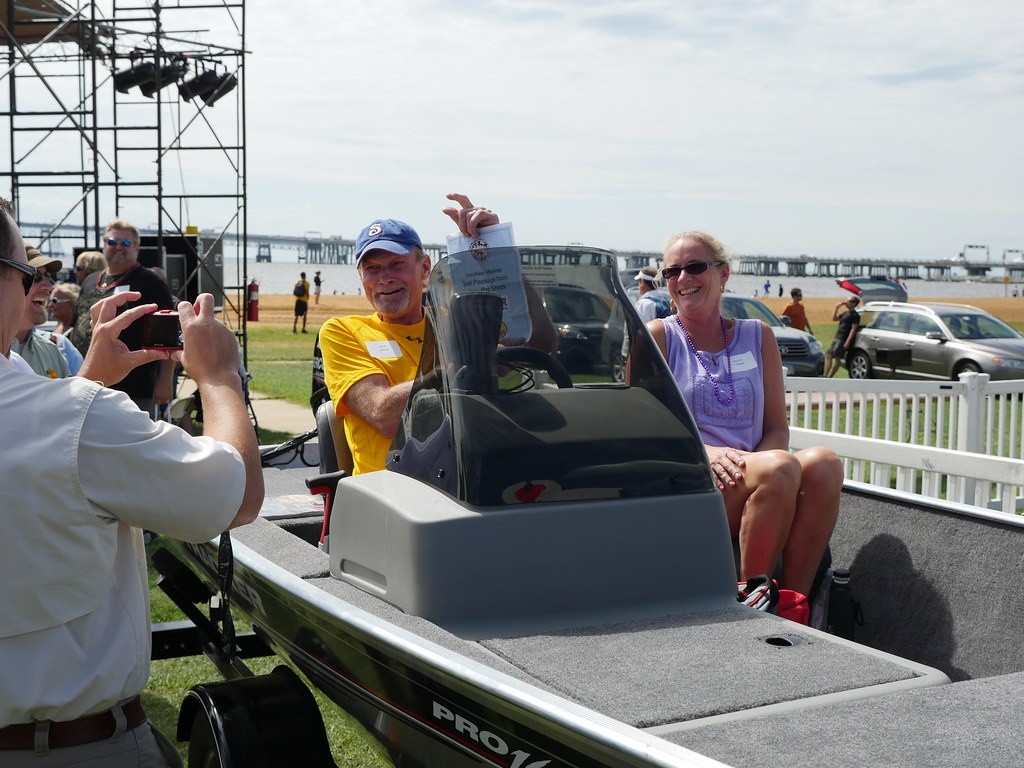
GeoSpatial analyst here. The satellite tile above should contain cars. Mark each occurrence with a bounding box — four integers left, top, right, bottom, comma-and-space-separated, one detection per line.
835, 276, 1024, 389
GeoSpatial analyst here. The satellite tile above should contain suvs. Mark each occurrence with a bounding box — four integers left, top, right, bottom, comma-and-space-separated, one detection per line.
619, 269, 825, 393
537, 283, 625, 363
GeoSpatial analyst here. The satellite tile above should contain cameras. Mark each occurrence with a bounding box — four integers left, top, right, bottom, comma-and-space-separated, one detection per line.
142, 309, 185, 351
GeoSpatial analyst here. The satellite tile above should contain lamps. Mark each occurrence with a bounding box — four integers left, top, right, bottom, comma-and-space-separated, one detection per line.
115, 51, 236, 107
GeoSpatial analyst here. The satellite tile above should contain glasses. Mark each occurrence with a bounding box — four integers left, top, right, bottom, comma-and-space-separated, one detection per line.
34, 270, 53, 283
0, 255, 36, 296
661, 260, 724, 279
104, 238, 136, 247
793, 294, 801, 297
48, 296, 75, 305
76, 265, 88, 271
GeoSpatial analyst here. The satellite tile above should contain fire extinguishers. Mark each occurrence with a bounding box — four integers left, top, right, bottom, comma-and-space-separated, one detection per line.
248, 276, 259, 321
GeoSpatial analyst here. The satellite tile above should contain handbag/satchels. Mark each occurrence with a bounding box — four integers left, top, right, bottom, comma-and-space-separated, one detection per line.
738, 574, 778, 613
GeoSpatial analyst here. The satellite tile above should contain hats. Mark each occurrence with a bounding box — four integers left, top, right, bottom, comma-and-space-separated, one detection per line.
634, 269, 656, 283
355, 218, 422, 269
25, 245, 62, 272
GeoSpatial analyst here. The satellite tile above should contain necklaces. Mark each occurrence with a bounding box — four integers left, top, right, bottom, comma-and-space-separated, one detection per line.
676, 315, 734, 404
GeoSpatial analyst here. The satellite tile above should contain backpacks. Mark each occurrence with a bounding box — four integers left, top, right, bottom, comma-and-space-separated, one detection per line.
645, 295, 671, 320
294, 282, 306, 297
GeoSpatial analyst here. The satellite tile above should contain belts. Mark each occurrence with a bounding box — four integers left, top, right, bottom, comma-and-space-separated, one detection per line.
0, 699, 148, 751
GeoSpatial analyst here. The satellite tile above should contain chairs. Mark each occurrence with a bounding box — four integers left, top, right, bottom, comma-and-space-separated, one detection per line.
316, 401, 355, 554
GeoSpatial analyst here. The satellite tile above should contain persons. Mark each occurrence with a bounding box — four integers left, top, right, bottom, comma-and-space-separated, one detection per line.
314, 271, 322, 304
1012, 285, 1018, 297
150, 266, 180, 310
625, 231, 844, 598
318, 193, 559, 476
10, 243, 83, 380
783, 288, 813, 335
71, 220, 177, 419
779, 284, 783, 297
48, 283, 81, 338
0, 196, 265, 768
74, 251, 106, 284
824, 296, 861, 377
293, 272, 310, 333
764, 280, 770, 296
621, 266, 677, 358
